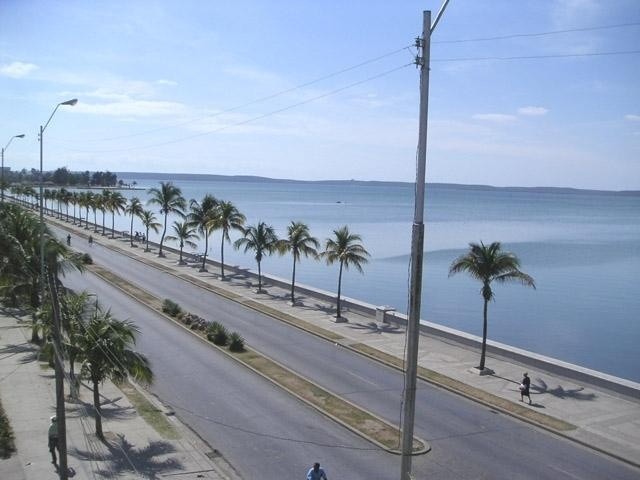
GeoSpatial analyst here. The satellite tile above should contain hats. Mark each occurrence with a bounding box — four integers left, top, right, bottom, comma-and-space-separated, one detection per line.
50, 415, 56, 421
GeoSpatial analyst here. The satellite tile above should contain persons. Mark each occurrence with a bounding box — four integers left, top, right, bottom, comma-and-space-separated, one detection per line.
134, 231, 146, 244
518, 371, 532, 405
306, 461, 328, 480
66, 234, 72, 246
46, 414, 60, 464
87, 234, 93, 248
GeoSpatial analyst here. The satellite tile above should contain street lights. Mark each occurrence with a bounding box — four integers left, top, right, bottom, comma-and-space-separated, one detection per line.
42, 96, 79, 480
0, 132, 25, 206
